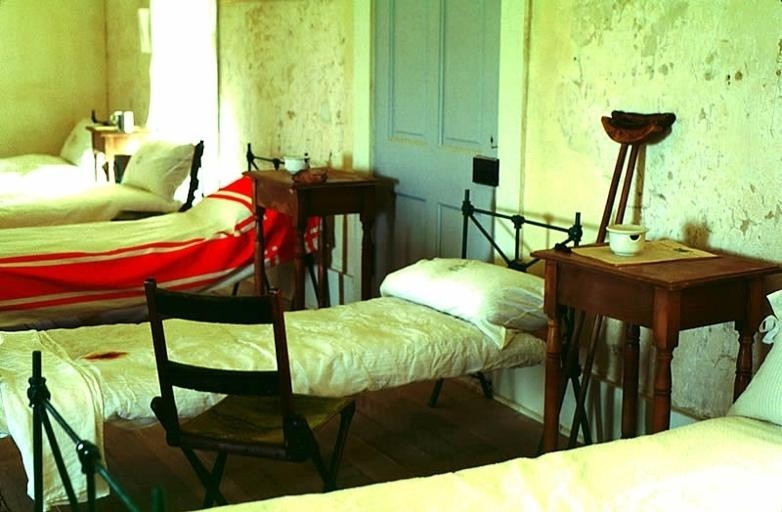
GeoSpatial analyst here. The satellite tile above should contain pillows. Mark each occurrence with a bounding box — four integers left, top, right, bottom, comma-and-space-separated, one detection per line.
378, 252, 549, 349
728, 319, 782, 425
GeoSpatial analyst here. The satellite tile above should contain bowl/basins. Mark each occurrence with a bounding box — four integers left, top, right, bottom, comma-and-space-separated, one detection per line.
606, 225, 649, 257
283, 155, 306, 174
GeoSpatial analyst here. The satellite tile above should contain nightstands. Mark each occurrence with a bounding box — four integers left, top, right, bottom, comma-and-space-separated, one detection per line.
242, 166, 398, 311
537, 238, 782, 460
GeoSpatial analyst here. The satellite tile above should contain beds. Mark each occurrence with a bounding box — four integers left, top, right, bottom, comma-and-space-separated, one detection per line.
0, 117, 324, 326
0, 193, 619, 443
195, 306, 782, 511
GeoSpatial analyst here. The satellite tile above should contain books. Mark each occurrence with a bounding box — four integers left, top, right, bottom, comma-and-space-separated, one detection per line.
568, 239, 721, 269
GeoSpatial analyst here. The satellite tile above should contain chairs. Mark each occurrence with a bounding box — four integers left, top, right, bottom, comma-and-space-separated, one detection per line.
142, 275, 357, 512
